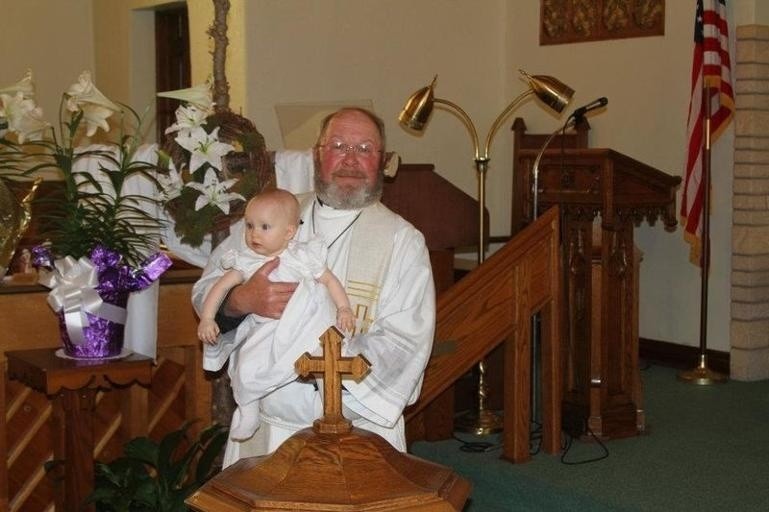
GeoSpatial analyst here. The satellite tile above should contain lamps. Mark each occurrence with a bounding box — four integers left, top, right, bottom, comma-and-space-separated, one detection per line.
400, 69, 576, 437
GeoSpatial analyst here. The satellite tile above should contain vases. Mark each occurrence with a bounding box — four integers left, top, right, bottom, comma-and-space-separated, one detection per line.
58, 270, 128, 356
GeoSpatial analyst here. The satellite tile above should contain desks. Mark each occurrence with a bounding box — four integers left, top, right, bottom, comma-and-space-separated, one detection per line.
4, 347, 154, 512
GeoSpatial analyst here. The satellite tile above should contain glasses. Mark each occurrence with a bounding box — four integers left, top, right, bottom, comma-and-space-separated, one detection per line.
316, 140, 380, 157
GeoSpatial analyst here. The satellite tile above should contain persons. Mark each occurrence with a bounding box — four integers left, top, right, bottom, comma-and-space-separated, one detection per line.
197, 188, 356, 442
194, 107, 436, 472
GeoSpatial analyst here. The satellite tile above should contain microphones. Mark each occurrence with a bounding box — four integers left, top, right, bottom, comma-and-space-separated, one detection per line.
571, 97, 609, 117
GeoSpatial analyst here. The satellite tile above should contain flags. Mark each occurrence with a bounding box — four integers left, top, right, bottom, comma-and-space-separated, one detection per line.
678, 0, 735, 268
